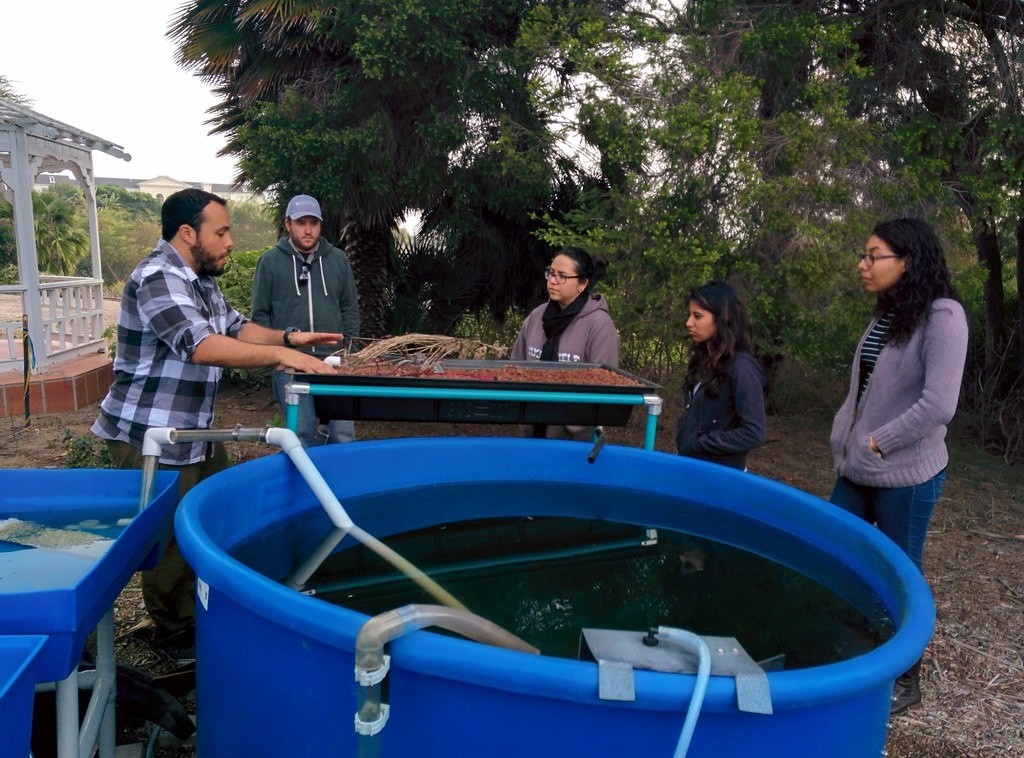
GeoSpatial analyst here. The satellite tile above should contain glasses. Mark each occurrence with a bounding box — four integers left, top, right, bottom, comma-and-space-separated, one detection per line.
544, 271, 579, 284
858, 253, 899, 266
298, 262, 311, 286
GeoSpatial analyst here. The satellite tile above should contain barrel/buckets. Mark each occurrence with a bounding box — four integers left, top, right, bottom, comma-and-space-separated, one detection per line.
174, 436, 937, 758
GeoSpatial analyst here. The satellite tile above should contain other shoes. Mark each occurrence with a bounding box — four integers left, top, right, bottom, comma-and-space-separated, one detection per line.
150, 617, 197, 650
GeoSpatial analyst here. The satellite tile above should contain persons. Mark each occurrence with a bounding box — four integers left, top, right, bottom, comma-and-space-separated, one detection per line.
251, 195, 358, 445
511, 246, 620, 443
676, 280, 769, 472
828, 216, 969, 573
88, 188, 344, 646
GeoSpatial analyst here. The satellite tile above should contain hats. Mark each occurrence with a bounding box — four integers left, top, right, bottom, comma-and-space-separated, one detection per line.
286, 194, 323, 221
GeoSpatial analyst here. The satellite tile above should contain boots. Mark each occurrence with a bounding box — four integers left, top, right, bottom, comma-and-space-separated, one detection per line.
888, 655, 924, 715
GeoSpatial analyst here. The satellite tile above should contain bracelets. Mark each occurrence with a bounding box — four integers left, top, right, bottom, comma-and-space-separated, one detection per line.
284, 326, 301, 348
